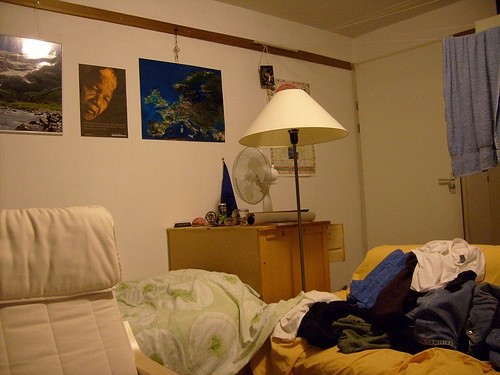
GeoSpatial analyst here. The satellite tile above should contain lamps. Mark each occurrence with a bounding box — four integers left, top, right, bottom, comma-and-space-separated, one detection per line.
236, 79, 350, 292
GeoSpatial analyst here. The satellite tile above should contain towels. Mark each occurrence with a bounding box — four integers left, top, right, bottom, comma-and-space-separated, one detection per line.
439, 28, 498, 177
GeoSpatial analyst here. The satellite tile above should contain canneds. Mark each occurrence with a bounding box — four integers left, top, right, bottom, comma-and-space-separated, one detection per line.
217, 203, 227, 222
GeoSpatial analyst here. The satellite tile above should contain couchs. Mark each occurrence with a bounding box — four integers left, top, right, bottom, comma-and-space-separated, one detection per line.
256, 243, 500, 375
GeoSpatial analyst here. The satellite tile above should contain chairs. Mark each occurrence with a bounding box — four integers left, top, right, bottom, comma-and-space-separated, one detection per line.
0, 204, 179, 375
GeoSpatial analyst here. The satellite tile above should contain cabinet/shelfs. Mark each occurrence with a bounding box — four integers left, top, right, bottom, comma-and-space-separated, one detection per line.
165, 221, 332, 304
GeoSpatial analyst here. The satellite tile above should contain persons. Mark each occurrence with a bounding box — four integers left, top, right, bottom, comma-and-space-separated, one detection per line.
80, 67, 117, 120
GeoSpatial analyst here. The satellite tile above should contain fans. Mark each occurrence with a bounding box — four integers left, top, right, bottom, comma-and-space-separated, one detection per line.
231, 147, 280, 213
